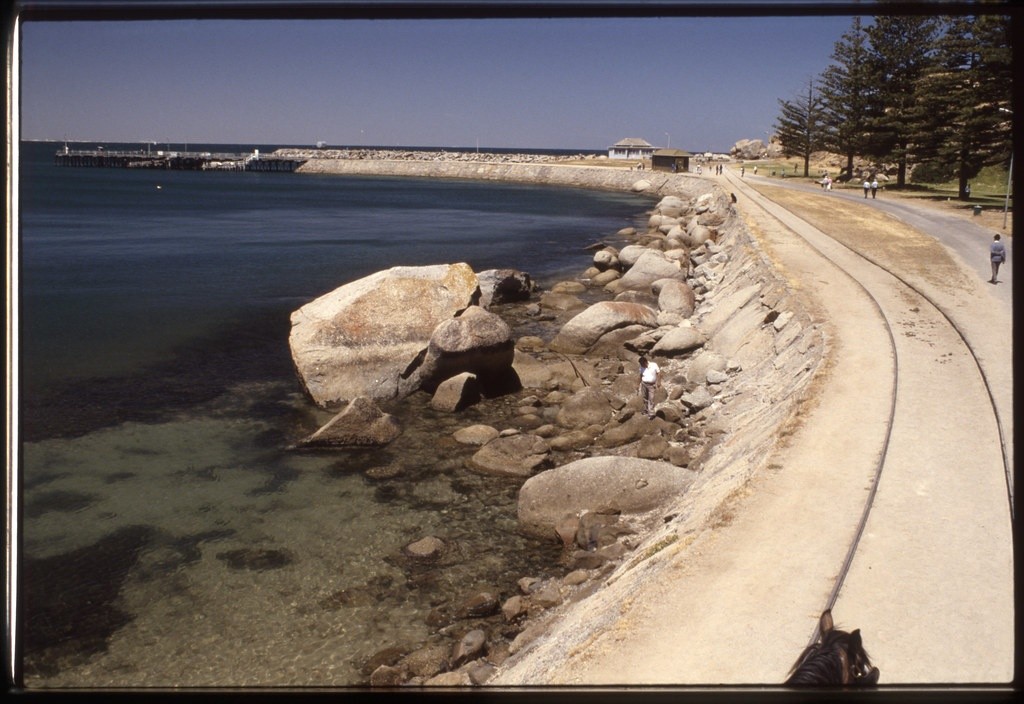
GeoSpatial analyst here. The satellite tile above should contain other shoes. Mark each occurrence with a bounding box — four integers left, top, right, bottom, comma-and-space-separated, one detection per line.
642, 411, 647, 415
987, 280, 997, 284
649, 416, 655, 420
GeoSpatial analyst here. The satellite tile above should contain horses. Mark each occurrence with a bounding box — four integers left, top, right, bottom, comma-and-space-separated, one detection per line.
780, 610, 873, 686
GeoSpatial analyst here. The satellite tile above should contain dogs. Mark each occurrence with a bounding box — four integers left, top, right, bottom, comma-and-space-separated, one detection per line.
731, 193, 737, 203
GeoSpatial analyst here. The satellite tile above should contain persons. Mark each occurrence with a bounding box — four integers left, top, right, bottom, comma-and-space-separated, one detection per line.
642, 155, 645, 169
863, 180, 870, 199
754, 165, 758, 175
715, 162, 724, 176
636, 356, 662, 420
987, 233, 1006, 285
741, 166, 745, 177
781, 169, 784, 179
965, 184, 971, 200
822, 175, 832, 192
871, 178, 879, 199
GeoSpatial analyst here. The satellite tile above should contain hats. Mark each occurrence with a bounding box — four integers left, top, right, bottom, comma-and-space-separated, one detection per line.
994, 234, 1000, 238
